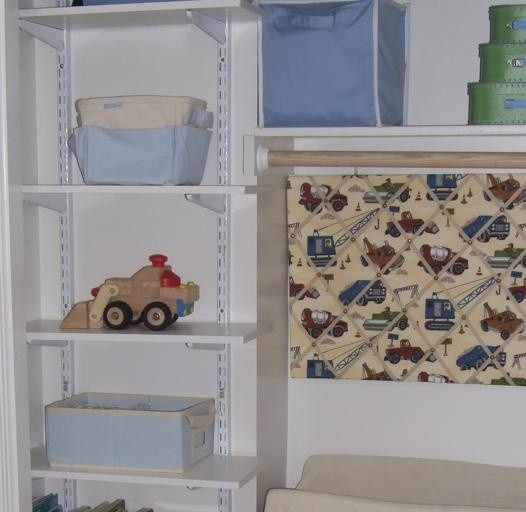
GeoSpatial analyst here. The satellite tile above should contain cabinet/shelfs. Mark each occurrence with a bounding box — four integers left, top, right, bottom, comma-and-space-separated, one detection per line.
0, 0, 286, 512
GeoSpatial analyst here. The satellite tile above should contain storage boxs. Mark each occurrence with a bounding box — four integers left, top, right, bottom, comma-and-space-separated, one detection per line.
489, 4, 526, 43
257, 0, 404, 127
467, 82, 526, 125
75, 95, 206, 130
44, 393, 215, 474
66, 126, 213, 185
479, 43, 526, 83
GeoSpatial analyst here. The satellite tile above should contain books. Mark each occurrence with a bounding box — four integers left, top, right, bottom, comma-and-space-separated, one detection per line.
31, 492, 153, 512
